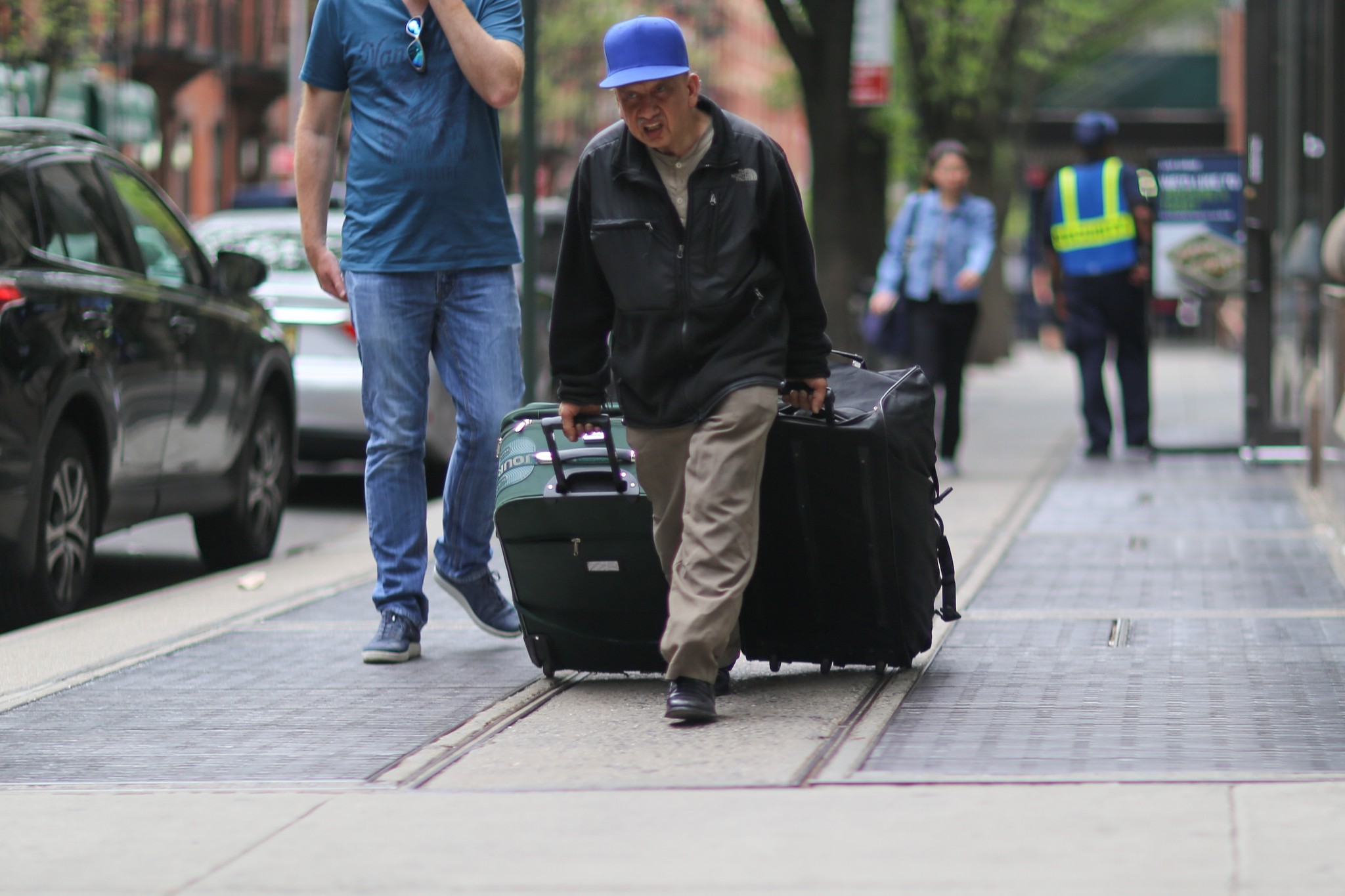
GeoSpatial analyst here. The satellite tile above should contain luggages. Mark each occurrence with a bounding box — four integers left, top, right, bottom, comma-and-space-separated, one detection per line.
738, 349, 954, 676
492, 401, 674, 677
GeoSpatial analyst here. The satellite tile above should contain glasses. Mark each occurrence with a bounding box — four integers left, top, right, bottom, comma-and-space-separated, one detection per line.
404, 13, 425, 73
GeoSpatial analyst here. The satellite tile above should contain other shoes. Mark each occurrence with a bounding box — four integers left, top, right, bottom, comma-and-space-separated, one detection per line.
1088, 440, 1107, 460
1128, 441, 1158, 464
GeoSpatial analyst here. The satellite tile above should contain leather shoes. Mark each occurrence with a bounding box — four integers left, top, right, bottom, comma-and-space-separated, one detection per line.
665, 674, 717, 719
712, 667, 729, 692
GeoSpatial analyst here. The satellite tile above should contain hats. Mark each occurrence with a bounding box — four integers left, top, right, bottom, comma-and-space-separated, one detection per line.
1076, 110, 1119, 147
598, 13, 690, 88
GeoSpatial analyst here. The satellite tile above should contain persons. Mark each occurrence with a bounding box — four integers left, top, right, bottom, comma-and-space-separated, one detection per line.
1038, 112, 1159, 459
295, 0, 526, 662
233, 140, 299, 208
548, 17, 829, 720
868, 139, 996, 475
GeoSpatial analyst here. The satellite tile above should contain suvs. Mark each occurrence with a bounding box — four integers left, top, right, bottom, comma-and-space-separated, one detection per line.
0, 109, 298, 634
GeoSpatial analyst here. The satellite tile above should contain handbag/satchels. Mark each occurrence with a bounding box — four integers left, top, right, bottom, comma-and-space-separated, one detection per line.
861, 295, 908, 350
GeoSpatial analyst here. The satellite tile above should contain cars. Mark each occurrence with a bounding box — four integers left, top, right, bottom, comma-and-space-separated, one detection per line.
185, 184, 570, 501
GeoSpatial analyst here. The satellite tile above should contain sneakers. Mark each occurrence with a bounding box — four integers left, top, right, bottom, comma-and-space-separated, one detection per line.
361, 607, 421, 664
434, 562, 522, 638
